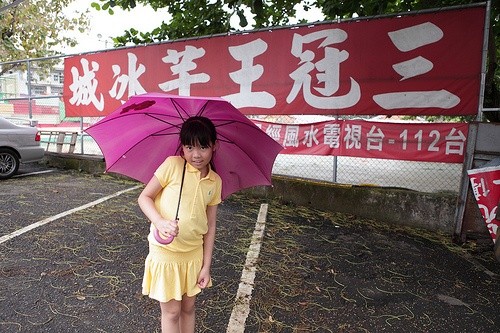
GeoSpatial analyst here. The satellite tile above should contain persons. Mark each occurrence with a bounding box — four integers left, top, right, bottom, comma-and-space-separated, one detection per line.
136, 117, 222, 333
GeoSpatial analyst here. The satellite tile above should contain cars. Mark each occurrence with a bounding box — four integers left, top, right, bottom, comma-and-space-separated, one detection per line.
0, 117, 45, 180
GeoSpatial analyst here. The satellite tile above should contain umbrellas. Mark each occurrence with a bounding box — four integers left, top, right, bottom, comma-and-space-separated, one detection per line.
83, 92, 286, 245
